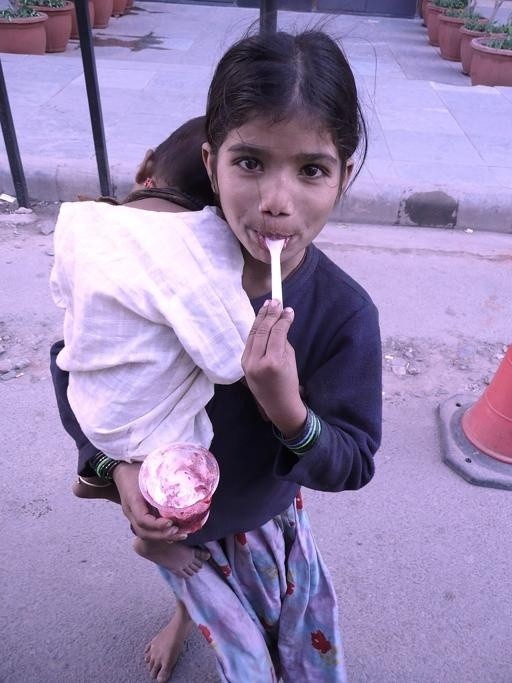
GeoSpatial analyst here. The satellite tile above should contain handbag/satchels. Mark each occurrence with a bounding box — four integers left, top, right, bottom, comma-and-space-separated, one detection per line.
88, 450, 123, 485
271, 401, 322, 457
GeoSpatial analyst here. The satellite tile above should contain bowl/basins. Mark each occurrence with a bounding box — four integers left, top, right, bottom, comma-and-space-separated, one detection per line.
124, 183, 202, 211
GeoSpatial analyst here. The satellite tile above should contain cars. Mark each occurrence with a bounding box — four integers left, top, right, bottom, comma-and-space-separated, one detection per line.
138, 444, 221, 535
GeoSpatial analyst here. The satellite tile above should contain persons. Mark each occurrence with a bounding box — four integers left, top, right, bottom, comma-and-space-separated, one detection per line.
46, 117, 260, 578
49, 28, 380, 680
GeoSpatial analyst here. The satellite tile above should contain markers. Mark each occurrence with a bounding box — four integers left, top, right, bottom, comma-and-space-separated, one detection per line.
148, 448, 213, 535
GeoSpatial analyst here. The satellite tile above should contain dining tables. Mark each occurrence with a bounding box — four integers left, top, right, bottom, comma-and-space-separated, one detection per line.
416, 0, 512, 87
0, 1, 133, 55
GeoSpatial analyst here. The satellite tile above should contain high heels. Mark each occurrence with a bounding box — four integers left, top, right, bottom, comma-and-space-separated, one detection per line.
437, 343, 512, 488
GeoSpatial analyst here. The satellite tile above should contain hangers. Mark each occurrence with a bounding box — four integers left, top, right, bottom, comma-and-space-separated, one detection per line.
264, 236, 286, 314
